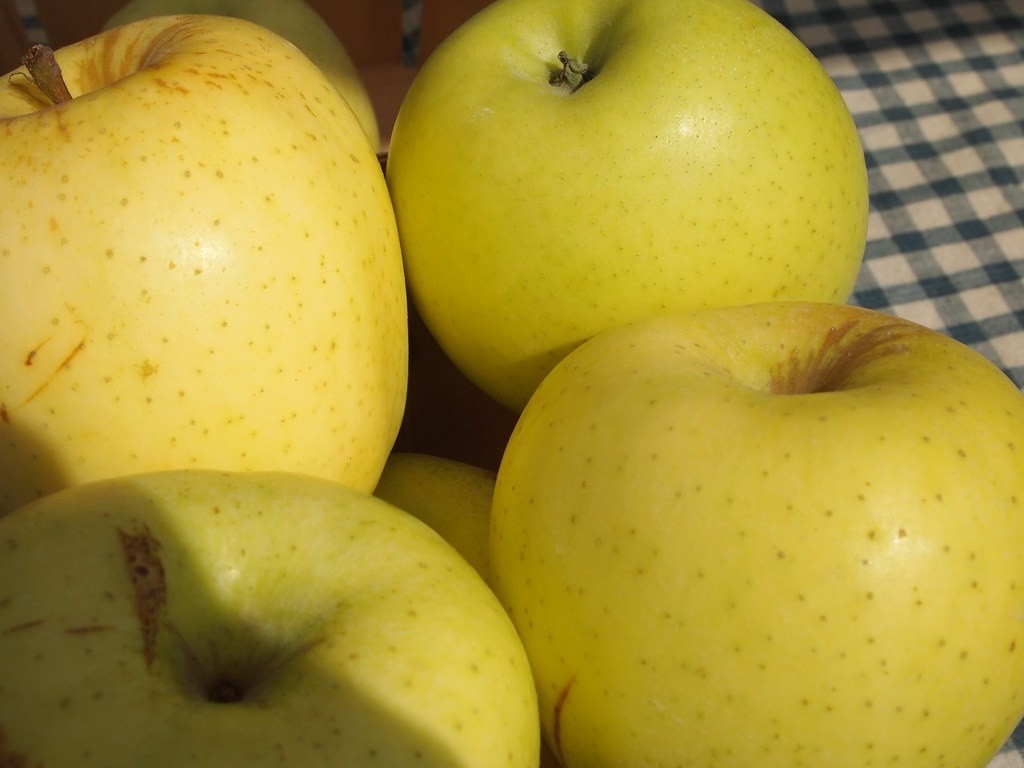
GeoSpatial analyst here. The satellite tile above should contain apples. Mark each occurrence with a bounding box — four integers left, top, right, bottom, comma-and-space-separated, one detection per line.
0, 0, 1024, 768
